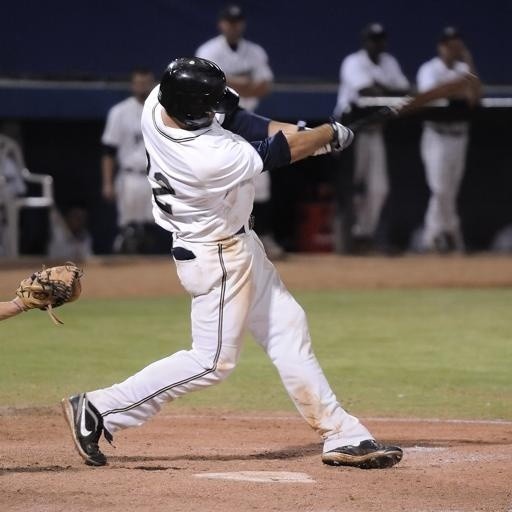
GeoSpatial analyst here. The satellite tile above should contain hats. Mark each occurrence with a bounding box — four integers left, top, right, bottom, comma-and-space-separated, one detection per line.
435, 26, 462, 45
367, 22, 388, 38
223, 5, 244, 22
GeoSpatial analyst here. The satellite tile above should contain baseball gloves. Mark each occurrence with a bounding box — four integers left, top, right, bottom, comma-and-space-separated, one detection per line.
16, 262, 80, 311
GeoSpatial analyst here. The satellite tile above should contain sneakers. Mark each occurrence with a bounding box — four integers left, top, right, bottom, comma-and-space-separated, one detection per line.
321, 436, 404, 470
59, 391, 112, 466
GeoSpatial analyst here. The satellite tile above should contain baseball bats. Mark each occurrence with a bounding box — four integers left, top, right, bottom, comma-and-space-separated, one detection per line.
330, 79, 458, 149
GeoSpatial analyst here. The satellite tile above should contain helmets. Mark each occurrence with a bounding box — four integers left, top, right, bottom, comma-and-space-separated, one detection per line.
156, 55, 243, 119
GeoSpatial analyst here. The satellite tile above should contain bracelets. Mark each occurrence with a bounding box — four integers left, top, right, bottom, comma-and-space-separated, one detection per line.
11, 299, 22, 312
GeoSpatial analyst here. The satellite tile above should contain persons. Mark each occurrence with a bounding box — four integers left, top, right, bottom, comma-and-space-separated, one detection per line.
1, 259, 80, 319
198, 4, 288, 260
45, 201, 96, 257
91, 59, 163, 254
62, 54, 404, 468
416, 27, 483, 254
329, 21, 412, 247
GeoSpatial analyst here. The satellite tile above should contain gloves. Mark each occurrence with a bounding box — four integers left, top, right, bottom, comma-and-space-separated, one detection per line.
330, 118, 355, 153
310, 142, 333, 157
